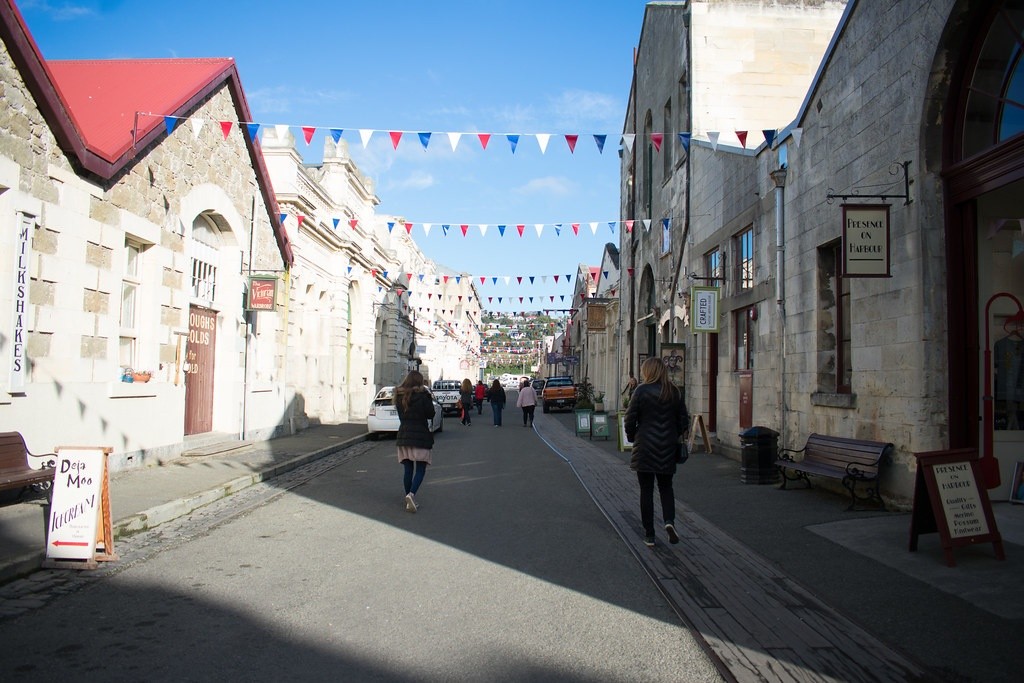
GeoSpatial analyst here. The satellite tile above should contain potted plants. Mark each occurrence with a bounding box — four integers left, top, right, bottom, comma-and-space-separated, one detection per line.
593, 391, 606, 412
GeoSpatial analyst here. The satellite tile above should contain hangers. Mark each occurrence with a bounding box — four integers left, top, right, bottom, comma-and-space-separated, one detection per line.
1007, 330, 1024, 340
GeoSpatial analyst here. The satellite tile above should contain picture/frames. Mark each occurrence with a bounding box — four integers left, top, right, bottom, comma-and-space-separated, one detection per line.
1008, 460, 1024, 504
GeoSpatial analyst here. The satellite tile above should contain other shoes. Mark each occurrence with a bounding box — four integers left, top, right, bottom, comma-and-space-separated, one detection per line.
461, 420, 465, 425
466, 423, 472, 426
494, 424, 498, 427
405, 492, 420, 513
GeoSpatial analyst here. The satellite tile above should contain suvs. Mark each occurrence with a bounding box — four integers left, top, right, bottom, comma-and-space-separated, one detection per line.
541, 374, 579, 414
529, 380, 545, 396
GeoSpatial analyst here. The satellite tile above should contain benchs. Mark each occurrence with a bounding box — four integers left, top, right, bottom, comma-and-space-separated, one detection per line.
0, 431, 58, 508
773, 432, 894, 513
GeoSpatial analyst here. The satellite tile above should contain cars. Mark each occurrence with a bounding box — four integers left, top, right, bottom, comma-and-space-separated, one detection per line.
367, 385, 445, 441
471, 385, 478, 403
483, 383, 490, 397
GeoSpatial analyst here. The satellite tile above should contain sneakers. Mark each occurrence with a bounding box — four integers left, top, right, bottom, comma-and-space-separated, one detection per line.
664, 519, 679, 544
643, 537, 655, 546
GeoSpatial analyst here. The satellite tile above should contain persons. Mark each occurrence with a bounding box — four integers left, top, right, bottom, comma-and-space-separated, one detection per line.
621, 372, 637, 400
516, 381, 538, 428
393, 370, 435, 512
624, 357, 688, 547
474, 380, 485, 415
487, 380, 506, 427
458, 379, 472, 426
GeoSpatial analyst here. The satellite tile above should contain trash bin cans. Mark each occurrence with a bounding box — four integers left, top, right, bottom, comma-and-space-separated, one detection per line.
738, 424, 782, 484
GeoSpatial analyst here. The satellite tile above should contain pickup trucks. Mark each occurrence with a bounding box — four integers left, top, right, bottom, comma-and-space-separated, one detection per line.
430, 379, 474, 417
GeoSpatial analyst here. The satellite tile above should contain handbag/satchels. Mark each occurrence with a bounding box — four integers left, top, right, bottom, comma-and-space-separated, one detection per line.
457, 399, 463, 409
473, 396, 478, 402
676, 421, 689, 463
503, 401, 506, 409
